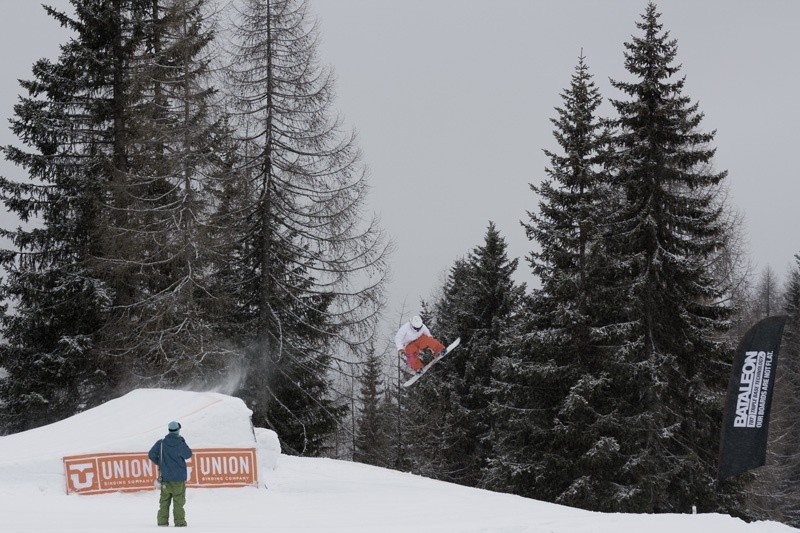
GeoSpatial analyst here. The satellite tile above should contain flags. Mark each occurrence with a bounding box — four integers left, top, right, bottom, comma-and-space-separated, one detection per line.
716, 315, 787, 474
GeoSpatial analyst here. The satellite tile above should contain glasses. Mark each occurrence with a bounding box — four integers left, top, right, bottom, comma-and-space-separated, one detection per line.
178, 422, 181, 428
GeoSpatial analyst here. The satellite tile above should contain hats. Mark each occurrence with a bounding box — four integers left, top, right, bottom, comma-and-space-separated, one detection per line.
168, 422, 179, 431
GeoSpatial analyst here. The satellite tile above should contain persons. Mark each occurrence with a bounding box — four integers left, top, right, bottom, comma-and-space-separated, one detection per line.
147, 421, 193, 528
395, 315, 447, 376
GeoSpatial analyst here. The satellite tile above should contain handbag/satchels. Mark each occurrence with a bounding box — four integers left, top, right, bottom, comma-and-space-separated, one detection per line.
155, 477, 162, 489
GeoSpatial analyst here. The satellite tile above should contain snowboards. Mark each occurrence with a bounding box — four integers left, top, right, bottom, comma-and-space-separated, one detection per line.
402, 337, 461, 388
